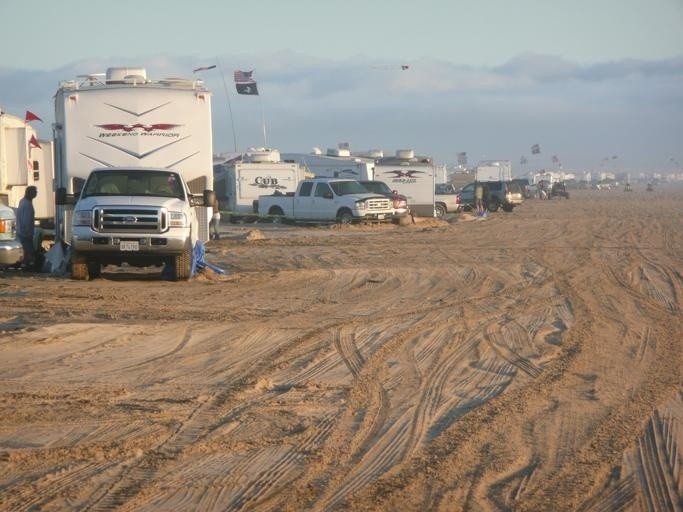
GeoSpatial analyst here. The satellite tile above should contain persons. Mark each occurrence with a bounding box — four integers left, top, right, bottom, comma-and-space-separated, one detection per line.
14, 184, 40, 265
209, 198, 221, 240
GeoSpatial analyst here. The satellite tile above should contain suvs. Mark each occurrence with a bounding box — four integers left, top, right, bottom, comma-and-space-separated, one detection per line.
356, 180, 408, 222
434, 179, 570, 217
0, 199, 41, 268
54, 166, 216, 282
567, 178, 622, 192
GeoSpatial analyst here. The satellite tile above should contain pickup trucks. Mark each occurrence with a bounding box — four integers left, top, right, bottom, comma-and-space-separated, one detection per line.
256, 176, 396, 225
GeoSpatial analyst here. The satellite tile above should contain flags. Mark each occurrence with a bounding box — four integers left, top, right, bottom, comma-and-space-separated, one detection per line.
232, 70, 254, 82
236, 84, 258, 95
